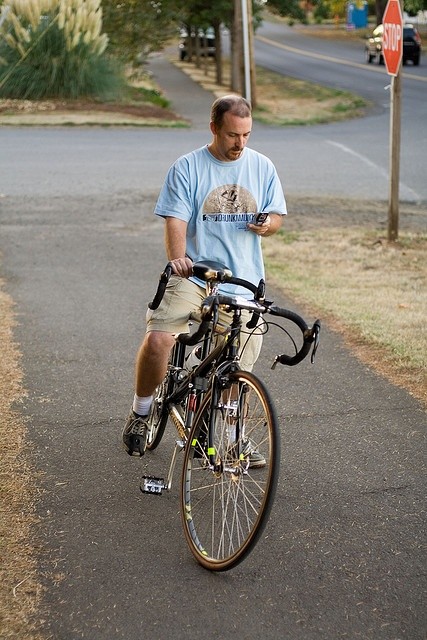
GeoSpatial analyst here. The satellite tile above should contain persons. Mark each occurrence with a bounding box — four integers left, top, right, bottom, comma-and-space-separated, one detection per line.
121, 93, 288, 468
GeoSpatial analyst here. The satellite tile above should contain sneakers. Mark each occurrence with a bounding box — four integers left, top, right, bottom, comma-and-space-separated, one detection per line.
225, 439, 267, 469
121, 399, 157, 458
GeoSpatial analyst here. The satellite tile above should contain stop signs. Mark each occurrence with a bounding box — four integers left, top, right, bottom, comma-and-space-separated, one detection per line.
380, 1, 404, 77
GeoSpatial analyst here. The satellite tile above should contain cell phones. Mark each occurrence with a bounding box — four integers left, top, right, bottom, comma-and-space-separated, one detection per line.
252, 212, 270, 232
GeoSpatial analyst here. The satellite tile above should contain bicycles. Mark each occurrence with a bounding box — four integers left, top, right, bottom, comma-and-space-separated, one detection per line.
136, 261, 320, 572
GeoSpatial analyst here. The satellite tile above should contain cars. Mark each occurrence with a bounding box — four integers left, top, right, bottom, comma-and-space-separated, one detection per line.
178, 25, 219, 62
362, 22, 421, 65
